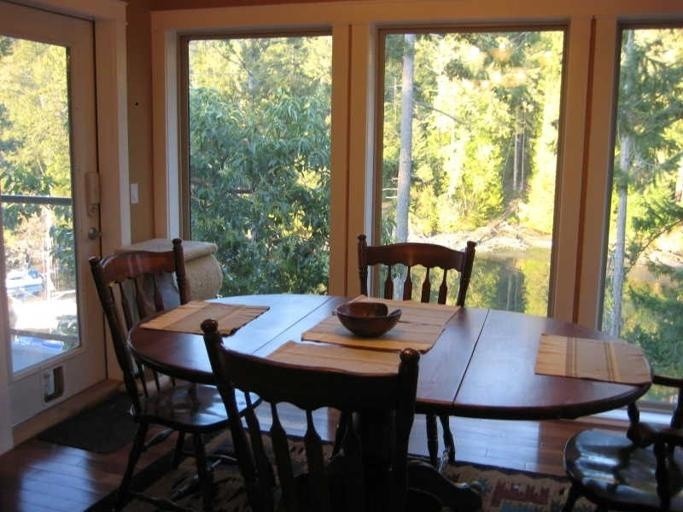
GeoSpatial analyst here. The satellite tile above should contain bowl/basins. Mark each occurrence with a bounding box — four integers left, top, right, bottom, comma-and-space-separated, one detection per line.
335, 302, 401, 338
